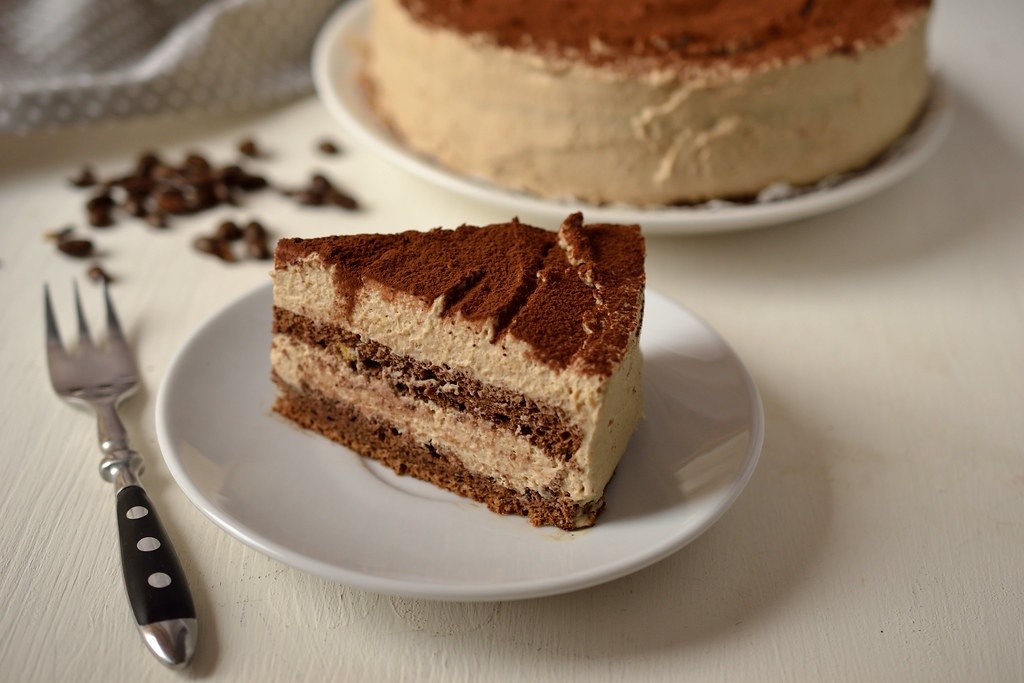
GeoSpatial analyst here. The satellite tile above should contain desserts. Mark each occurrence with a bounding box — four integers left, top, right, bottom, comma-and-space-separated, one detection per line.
268, 212, 646, 531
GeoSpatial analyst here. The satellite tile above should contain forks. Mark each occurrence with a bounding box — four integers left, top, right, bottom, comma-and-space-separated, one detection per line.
41, 280, 200, 670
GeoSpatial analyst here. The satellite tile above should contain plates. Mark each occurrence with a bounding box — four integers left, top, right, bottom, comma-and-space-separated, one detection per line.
311, 0, 961, 237
155, 283, 763, 597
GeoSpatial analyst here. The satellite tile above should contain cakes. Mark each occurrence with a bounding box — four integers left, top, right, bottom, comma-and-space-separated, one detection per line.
367, 0, 934, 209
